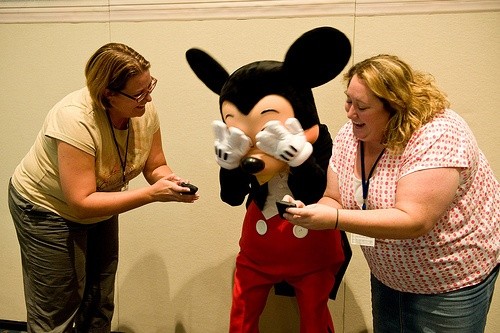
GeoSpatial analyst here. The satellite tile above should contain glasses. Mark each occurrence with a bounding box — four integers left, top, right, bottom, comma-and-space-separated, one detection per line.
118, 76, 157, 103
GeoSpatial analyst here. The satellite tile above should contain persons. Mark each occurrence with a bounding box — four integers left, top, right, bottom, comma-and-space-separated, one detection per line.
281, 54, 500, 333
8, 42, 199, 333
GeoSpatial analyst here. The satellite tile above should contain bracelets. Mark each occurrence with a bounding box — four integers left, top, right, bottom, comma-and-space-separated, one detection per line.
335, 210, 338, 230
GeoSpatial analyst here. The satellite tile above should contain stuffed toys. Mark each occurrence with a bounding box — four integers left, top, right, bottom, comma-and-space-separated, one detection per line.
186, 27, 353, 333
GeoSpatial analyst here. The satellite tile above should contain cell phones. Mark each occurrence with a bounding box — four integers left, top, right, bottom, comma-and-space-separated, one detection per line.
276, 201, 296, 219
180, 182, 198, 195
